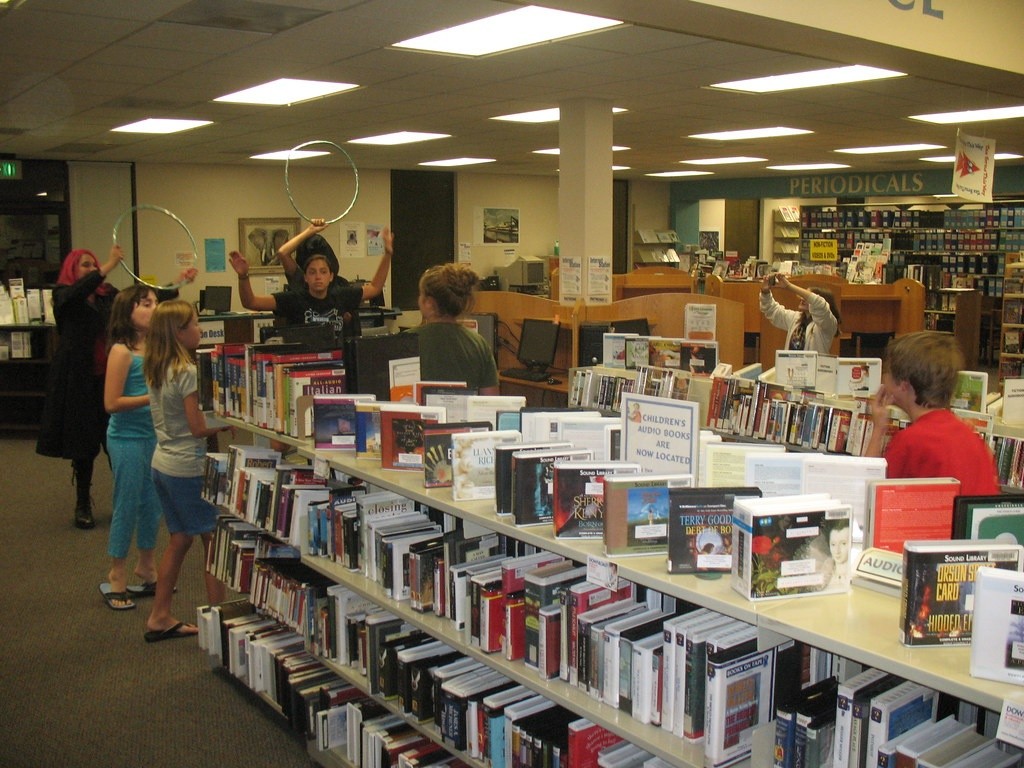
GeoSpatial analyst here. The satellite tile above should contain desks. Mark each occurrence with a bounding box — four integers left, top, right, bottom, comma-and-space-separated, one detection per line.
468, 268, 926, 393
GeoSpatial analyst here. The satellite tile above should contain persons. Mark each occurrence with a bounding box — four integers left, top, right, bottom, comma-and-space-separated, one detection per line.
35, 246, 196, 529
228, 227, 394, 342
404, 264, 498, 395
760, 273, 842, 354
98, 285, 177, 609
143, 301, 237, 642
864, 332, 1000, 495
277, 218, 361, 335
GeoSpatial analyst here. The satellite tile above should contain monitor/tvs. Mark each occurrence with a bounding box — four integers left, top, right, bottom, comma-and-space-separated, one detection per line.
517, 318, 561, 373
369, 289, 385, 307
199, 286, 232, 315
505, 255, 547, 286
612, 318, 649, 337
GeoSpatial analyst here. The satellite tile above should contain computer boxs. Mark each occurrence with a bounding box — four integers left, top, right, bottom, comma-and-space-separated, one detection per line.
468, 311, 498, 370
579, 320, 609, 367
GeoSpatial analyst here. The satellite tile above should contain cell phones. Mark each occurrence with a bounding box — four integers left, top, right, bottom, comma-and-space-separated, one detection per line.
769, 276, 776, 285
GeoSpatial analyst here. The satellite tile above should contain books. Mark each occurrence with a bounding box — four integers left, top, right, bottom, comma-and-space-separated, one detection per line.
199, 346, 1024, 768
639, 228, 922, 286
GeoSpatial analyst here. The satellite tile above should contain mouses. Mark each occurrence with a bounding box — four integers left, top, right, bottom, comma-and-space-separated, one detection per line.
549, 379, 562, 385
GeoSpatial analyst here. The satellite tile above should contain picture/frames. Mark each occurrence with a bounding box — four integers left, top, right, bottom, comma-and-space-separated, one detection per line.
238, 217, 302, 275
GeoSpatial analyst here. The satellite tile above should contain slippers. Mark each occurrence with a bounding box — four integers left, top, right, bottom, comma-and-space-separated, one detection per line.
99, 583, 134, 608
129, 582, 176, 593
144, 622, 199, 641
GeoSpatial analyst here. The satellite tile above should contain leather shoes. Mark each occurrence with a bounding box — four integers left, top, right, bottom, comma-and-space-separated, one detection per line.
75, 510, 93, 528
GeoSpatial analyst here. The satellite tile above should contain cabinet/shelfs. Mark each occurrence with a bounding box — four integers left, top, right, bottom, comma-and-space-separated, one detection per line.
632, 241, 680, 267
0, 324, 58, 437
206, 409, 1024, 768
771, 210, 800, 263
798, 200, 1024, 331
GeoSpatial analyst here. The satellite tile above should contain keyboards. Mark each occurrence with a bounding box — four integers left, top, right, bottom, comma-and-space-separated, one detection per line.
500, 368, 549, 382
